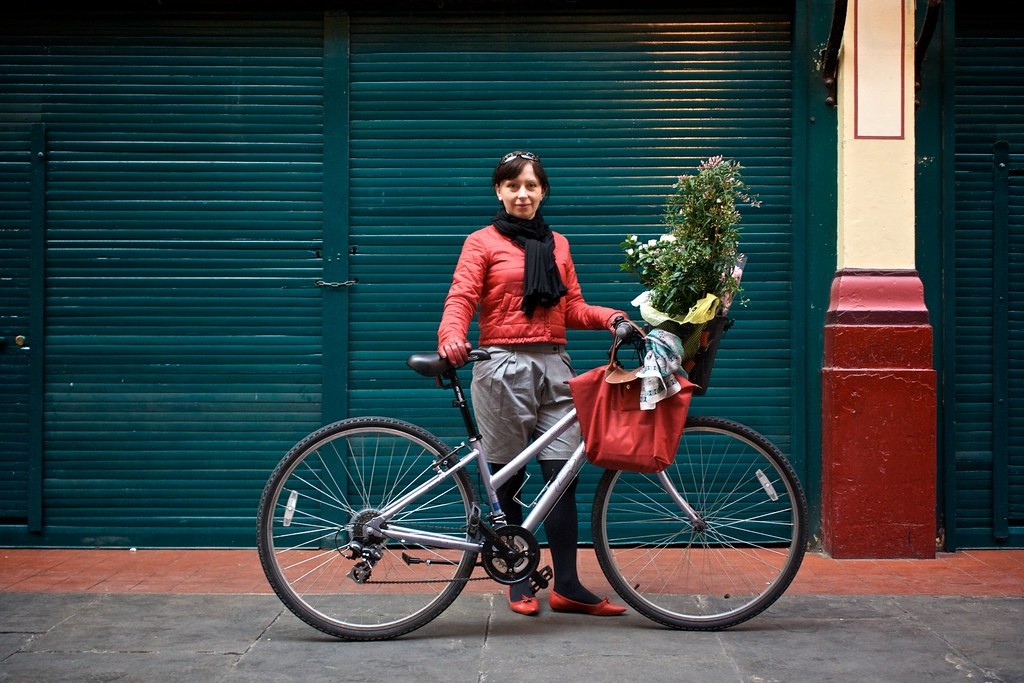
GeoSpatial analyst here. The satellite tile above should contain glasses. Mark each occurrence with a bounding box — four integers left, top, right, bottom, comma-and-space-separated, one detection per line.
495, 150, 541, 174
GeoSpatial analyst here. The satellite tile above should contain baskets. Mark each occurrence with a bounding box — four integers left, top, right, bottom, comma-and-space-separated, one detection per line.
648, 312, 728, 396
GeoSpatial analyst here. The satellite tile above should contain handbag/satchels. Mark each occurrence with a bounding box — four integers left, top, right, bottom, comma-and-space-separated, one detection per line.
567, 320, 702, 472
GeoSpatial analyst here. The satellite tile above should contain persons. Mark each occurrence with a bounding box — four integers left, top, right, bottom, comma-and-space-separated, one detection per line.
437, 151, 630, 614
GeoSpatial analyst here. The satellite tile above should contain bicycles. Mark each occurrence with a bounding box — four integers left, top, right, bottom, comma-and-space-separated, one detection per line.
256, 316, 808, 642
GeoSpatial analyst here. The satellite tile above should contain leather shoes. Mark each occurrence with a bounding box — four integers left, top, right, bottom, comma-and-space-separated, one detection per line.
506, 582, 540, 615
550, 588, 627, 615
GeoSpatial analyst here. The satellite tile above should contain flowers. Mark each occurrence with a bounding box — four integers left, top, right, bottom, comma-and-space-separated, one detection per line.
621, 155, 763, 372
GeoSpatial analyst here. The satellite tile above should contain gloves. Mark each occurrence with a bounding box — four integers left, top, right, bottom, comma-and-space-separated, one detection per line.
606, 313, 630, 335
439, 338, 473, 368
